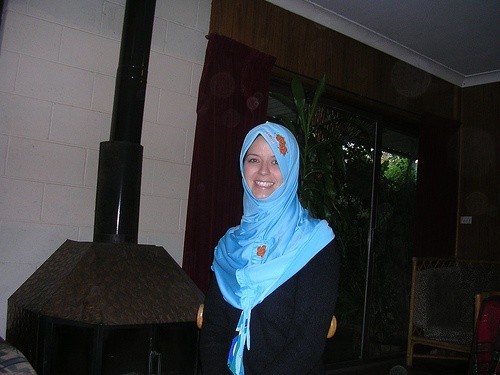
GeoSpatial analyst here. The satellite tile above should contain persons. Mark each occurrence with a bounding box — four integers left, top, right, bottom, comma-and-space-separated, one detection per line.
198, 122, 341, 375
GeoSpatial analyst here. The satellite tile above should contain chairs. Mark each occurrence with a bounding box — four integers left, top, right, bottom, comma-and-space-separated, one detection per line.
405, 257, 500, 369
